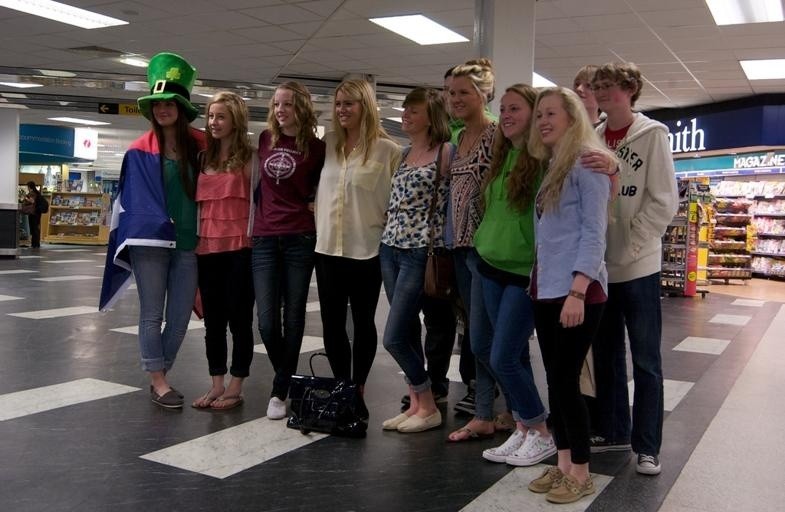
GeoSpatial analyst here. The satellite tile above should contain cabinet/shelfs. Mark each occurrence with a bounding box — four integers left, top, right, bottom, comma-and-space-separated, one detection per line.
47, 192, 110, 245
706, 195, 754, 285
18, 172, 45, 247
676, 170, 784, 281
660, 177, 716, 300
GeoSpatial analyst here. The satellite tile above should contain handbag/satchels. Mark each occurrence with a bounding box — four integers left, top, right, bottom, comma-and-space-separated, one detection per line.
22, 197, 35, 215
424, 141, 458, 302
286, 352, 369, 439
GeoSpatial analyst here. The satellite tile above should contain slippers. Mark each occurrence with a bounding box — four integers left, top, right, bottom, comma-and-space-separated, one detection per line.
445, 427, 495, 444
493, 417, 515, 432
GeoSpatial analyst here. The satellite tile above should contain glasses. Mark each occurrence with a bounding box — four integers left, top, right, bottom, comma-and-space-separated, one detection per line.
590, 81, 622, 93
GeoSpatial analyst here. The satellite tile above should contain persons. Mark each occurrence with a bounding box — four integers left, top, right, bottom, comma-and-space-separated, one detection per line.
399, 65, 497, 414
447, 57, 515, 443
97, 53, 207, 407
312, 75, 403, 399
543, 65, 602, 431
256, 78, 325, 420
589, 60, 678, 478
24, 180, 42, 251
192, 92, 257, 412
528, 86, 610, 503
378, 87, 457, 431
472, 82, 622, 468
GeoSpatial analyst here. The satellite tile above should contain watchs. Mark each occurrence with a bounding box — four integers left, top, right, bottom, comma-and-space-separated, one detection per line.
607, 161, 622, 176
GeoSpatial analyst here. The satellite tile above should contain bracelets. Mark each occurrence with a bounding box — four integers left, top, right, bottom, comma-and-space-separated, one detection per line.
567, 288, 586, 300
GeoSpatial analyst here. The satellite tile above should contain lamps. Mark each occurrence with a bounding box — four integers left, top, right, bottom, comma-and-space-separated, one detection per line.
368, 14, 471, 45
533, 71, 557, 87
48, 117, 111, 125
1, 1, 130, 31
0, 81, 44, 89
741, 57, 785, 81
118, 58, 150, 68
705, 0, 785, 27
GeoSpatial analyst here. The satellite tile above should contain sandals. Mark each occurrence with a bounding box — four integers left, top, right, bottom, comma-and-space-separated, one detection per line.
211, 390, 243, 409
192, 386, 225, 407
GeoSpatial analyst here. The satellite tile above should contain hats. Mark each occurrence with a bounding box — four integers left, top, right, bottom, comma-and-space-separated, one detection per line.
137, 52, 199, 122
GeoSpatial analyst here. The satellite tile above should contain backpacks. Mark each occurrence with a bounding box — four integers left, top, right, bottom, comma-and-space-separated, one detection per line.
35, 191, 49, 214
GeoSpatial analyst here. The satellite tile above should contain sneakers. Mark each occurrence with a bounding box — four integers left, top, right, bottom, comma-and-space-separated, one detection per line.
589, 436, 632, 453
505, 427, 557, 466
150, 384, 184, 399
382, 413, 409, 430
397, 409, 442, 433
267, 397, 287, 419
401, 391, 448, 405
151, 389, 184, 408
546, 473, 596, 503
528, 465, 563, 493
482, 429, 526, 463
636, 451, 661, 475
454, 387, 476, 415
